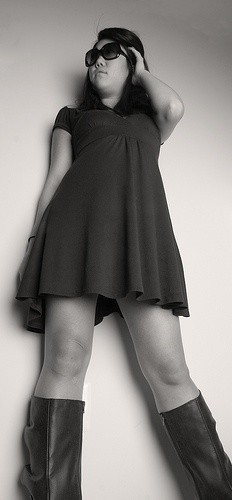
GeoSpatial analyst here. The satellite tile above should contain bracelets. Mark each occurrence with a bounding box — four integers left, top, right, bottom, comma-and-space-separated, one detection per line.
28, 236, 36, 241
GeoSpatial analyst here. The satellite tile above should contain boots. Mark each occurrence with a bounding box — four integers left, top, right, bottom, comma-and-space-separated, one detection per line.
158, 388, 232, 500
21, 395, 85, 499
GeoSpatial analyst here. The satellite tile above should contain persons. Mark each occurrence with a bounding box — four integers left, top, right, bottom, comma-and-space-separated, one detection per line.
15, 26, 231, 500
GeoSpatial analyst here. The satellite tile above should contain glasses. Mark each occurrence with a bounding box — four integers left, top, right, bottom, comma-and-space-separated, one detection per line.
84, 43, 134, 70
